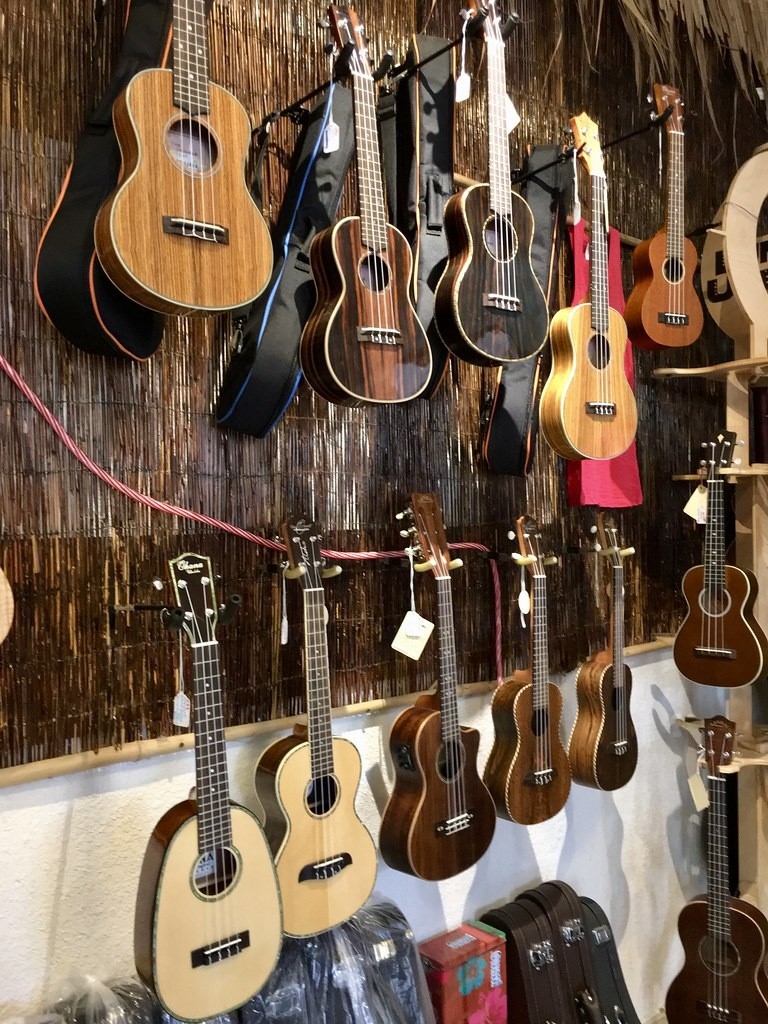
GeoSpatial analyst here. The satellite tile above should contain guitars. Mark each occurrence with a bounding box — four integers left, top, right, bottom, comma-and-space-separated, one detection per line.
252, 512, 377, 940
91, 1, 275, 316
567, 510, 638, 792
672, 428, 767, 689
378, 488, 496, 881
298, 3, 433, 408
664, 713, 768, 1024
537, 111, 639, 462
131, 550, 284, 1024
481, 514, 571, 826
432, 0, 550, 367
622, 83, 705, 350
